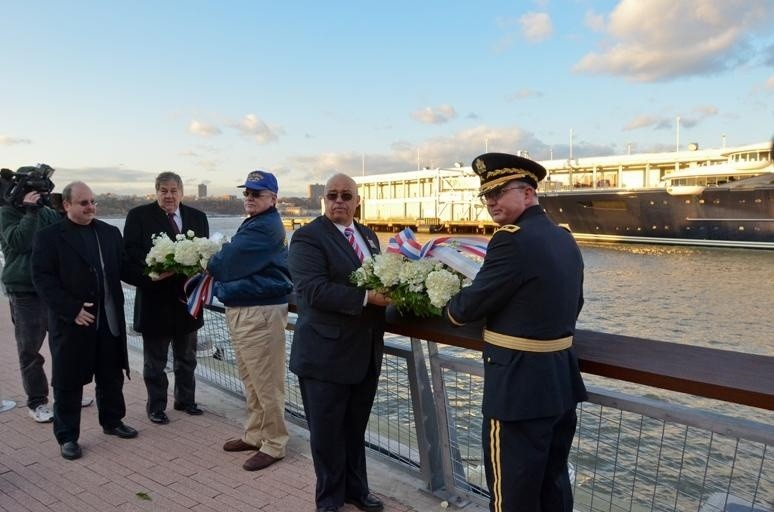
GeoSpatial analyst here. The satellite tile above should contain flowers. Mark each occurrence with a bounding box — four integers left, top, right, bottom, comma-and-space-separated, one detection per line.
145, 230, 230, 277
348, 241, 476, 316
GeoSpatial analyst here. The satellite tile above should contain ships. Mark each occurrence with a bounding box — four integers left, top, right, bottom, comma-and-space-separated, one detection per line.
536, 142, 774, 251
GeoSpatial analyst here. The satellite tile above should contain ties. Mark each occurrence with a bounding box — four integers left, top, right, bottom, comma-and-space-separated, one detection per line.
168, 212, 180, 235
344, 227, 365, 265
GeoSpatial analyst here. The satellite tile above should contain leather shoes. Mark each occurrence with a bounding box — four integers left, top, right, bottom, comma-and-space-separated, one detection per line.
174, 404, 202, 415
103, 420, 137, 438
147, 410, 169, 424
344, 492, 384, 511
60, 439, 81, 459
243, 451, 285, 471
223, 438, 260, 452
317, 505, 338, 512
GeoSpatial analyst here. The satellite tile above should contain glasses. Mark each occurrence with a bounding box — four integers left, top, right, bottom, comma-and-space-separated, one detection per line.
79, 199, 98, 207
480, 186, 524, 205
326, 192, 353, 201
242, 191, 272, 199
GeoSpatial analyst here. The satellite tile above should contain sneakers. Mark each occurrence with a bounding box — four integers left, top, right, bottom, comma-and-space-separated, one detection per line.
27, 405, 54, 423
82, 399, 93, 407
0, 400, 16, 412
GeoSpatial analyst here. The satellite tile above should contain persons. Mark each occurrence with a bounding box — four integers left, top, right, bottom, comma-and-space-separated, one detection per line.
288, 172, 394, 512
206, 171, 292, 472
442, 153, 589, 512
124, 171, 209, 423
30, 181, 175, 460
0, 166, 94, 424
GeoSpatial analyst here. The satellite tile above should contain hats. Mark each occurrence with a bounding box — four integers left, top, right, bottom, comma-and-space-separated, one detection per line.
471, 152, 547, 198
236, 170, 278, 194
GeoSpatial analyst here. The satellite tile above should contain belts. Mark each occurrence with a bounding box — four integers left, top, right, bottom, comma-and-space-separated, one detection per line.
481, 328, 574, 354
